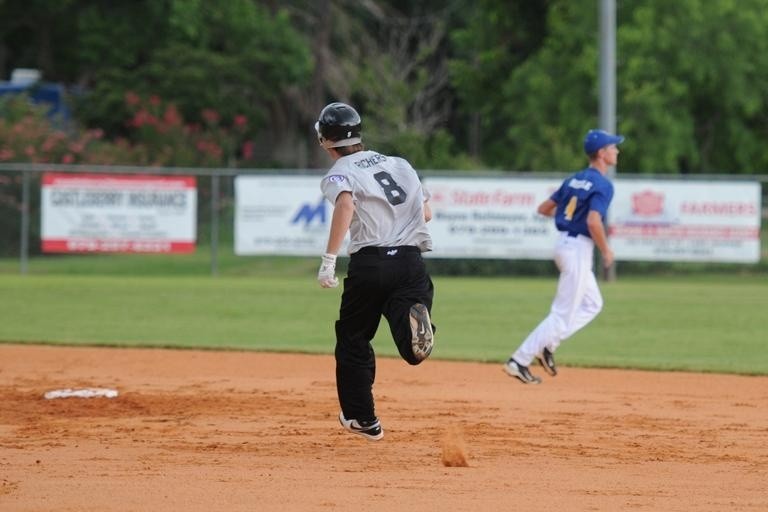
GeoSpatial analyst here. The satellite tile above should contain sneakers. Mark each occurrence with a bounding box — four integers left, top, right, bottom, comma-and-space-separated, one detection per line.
408, 301, 436, 362
501, 355, 543, 386
536, 345, 558, 377
338, 408, 385, 441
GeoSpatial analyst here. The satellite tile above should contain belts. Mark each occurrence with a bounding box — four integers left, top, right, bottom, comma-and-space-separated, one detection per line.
566, 229, 580, 238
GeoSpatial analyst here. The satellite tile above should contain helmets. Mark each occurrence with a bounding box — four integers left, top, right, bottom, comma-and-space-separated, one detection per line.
316, 101, 365, 149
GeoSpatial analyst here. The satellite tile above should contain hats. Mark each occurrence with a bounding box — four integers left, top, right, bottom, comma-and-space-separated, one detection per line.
584, 128, 628, 154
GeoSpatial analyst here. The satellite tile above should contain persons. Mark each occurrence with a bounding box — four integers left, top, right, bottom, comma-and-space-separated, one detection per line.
500, 128, 628, 383
313, 103, 436, 443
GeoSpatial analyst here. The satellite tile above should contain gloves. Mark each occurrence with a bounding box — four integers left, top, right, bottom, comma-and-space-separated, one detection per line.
317, 252, 341, 290
